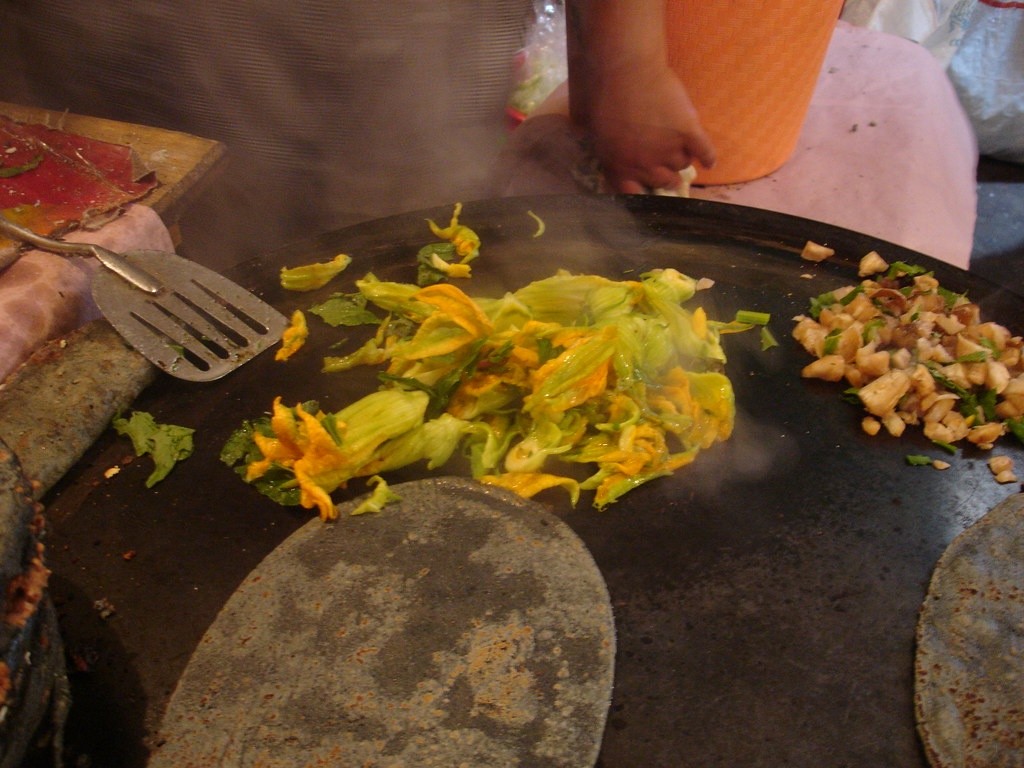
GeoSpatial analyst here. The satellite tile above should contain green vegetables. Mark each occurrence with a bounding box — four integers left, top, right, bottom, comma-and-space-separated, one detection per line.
737, 261, 1024, 468
107, 198, 771, 524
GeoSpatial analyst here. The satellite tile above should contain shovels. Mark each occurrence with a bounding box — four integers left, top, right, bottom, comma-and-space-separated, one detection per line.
0, 219, 292, 384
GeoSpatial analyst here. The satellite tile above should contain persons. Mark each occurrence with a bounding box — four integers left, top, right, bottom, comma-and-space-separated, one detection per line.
0, 0, 715, 276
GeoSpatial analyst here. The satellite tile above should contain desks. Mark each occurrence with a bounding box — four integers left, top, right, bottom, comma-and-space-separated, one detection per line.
3, 100, 226, 395
